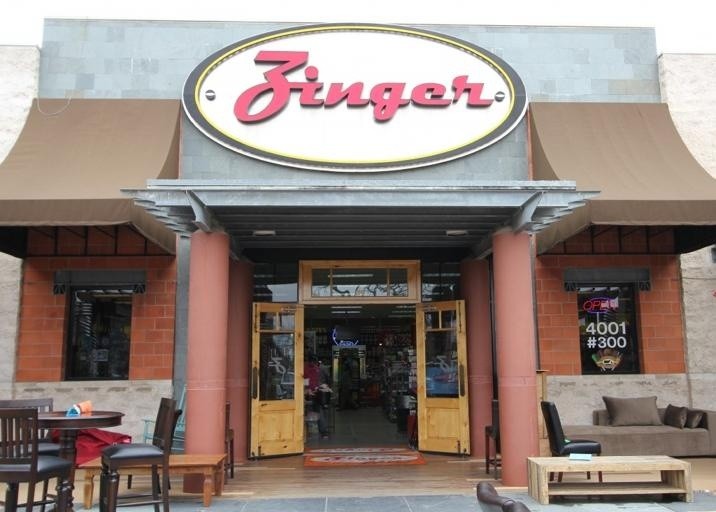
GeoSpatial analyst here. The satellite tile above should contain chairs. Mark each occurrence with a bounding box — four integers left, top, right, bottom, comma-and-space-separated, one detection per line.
541, 400, 603, 502
94, 394, 179, 510
473, 480, 530, 511
223, 404, 235, 480
481, 397, 504, 478
126, 411, 181, 490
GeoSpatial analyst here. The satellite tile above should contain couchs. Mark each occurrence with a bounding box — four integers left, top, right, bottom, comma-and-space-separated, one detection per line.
534, 409, 715, 460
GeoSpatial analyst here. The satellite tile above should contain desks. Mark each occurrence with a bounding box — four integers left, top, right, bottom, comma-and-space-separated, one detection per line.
26, 411, 126, 510
78, 449, 227, 507
526, 456, 693, 503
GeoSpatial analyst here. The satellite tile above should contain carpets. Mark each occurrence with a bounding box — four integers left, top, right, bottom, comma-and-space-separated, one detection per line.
302, 442, 426, 470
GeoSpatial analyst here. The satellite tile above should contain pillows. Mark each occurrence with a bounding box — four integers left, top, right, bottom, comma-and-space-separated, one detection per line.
602, 393, 663, 429
686, 410, 702, 427
664, 405, 689, 431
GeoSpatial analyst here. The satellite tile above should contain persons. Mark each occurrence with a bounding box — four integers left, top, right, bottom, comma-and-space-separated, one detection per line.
305, 355, 327, 439
321, 359, 330, 410
